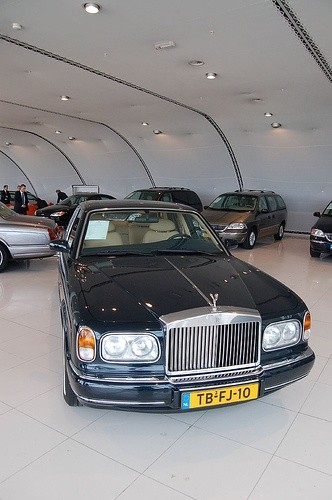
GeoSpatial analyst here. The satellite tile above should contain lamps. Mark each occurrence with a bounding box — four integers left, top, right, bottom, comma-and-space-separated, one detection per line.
264, 113, 271, 116
84, 3, 100, 14
206, 72, 216, 80
60, 96, 69, 101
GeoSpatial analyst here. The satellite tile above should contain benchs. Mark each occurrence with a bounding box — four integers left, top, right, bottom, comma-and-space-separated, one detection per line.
109, 222, 152, 245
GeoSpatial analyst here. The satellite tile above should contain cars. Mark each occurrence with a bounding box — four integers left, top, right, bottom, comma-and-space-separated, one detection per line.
0, 191, 48, 210
309, 200, 332, 260
48, 199, 316, 415
33, 192, 118, 229
0, 201, 63, 273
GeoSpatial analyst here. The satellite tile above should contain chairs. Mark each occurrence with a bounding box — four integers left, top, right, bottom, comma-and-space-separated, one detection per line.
163, 196, 169, 202
147, 196, 153, 200
84, 221, 123, 247
226, 197, 241, 209
143, 219, 181, 244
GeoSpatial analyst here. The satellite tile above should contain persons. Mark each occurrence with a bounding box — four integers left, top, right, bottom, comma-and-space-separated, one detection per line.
16, 186, 21, 190
56, 190, 71, 205
14, 184, 29, 215
1, 184, 11, 204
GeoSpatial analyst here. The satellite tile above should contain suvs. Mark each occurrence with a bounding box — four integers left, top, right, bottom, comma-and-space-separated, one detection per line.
123, 186, 203, 221
192, 189, 288, 249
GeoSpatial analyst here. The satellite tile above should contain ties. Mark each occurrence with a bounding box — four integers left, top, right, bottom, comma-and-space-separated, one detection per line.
21, 192, 24, 205
